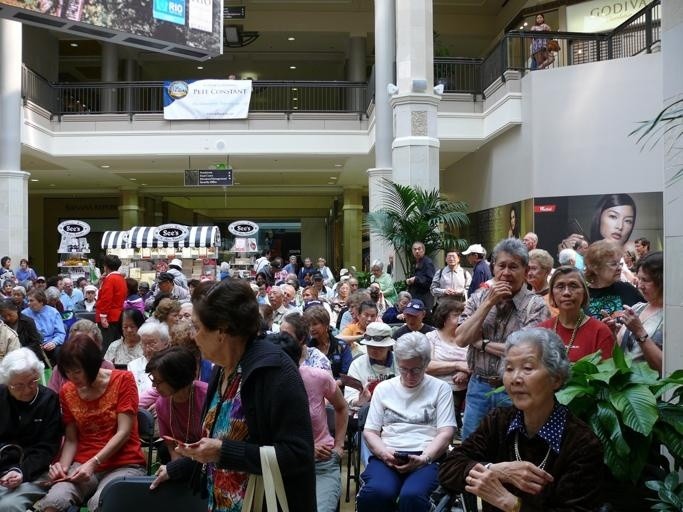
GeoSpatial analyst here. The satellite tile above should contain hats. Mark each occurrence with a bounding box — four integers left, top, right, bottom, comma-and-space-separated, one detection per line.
461, 244, 485, 256
313, 273, 323, 281
152, 272, 174, 283
35, 275, 45, 282
360, 322, 396, 347
84, 285, 99, 301
403, 299, 425, 317
167, 259, 183, 270
139, 282, 150, 289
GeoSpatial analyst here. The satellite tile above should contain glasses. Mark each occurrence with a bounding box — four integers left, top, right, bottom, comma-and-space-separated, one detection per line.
397, 366, 424, 375
148, 373, 165, 387
603, 261, 623, 268
10, 377, 41, 391
406, 302, 423, 311
364, 334, 392, 342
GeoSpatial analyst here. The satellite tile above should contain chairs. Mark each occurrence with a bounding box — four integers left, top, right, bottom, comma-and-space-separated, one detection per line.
137, 408, 166, 475
325, 374, 370, 502
96, 477, 207, 512
75, 312, 96, 323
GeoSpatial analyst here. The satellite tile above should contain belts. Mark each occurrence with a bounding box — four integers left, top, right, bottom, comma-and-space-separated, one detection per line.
479, 375, 502, 387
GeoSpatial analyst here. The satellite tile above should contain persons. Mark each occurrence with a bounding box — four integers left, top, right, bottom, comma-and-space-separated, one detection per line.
529, 13, 555, 69
590, 193, 636, 246
508, 207, 519, 239
0, 230, 663, 512
